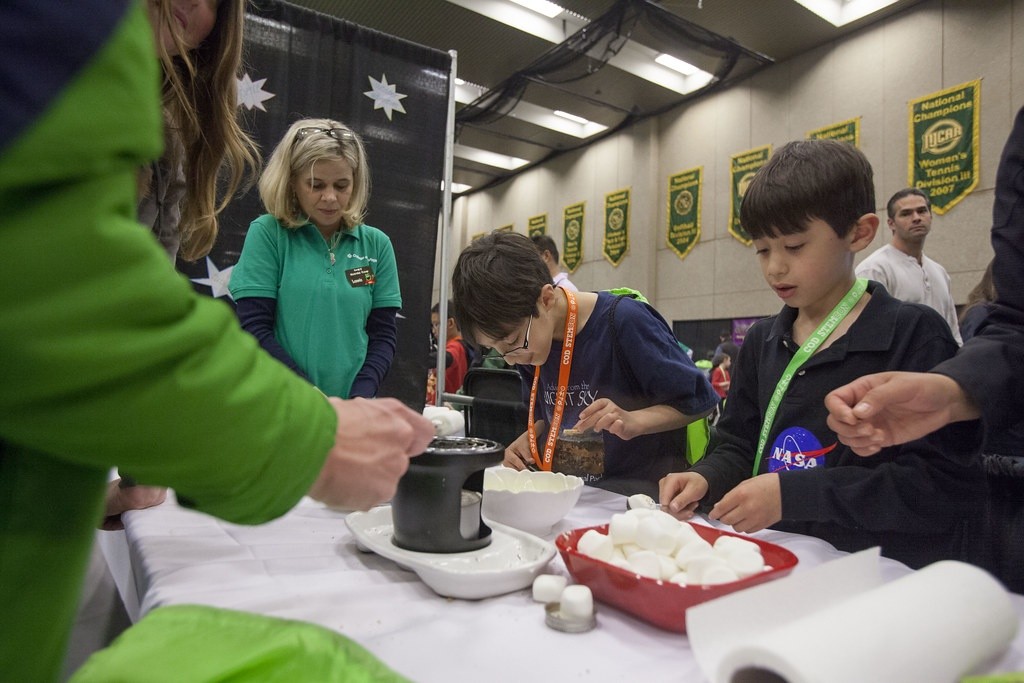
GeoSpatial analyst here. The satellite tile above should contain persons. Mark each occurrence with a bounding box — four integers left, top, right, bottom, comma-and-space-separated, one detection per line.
0, 0, 436, 683
852, 186, 963, 346
530, 236, 578, 292
454, 344, 541, 421
958, 256, 997, 340
453, 230, 719, 502
824, 103, 1024, 459
712, 330, 740, 380
428, 299, 483, 395
709, 352, 732, 426
658, 138, 981, 568
228, 117, 403, 401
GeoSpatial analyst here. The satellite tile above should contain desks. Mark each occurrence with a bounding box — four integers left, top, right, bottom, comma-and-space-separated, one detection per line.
122, 467, 1024, 683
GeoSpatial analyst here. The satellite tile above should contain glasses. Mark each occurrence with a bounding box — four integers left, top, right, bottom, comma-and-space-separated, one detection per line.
481, 283, 558, 361
292, 127, 357, 152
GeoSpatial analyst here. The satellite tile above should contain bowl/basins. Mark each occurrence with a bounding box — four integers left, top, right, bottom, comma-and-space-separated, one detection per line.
554, 521, 799, 635
482, 465, 585, 538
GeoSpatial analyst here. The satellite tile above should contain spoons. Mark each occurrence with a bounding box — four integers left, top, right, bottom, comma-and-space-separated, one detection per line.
627, 494, 700, 514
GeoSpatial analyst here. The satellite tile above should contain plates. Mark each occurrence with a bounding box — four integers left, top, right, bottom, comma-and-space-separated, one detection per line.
343, 505, 558, 601
